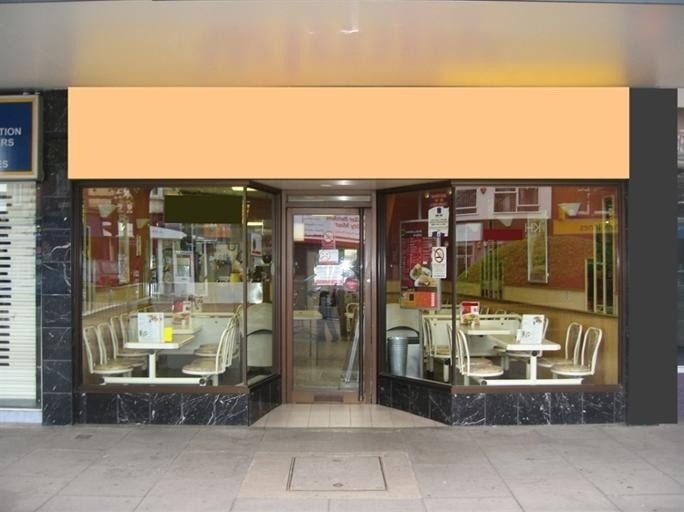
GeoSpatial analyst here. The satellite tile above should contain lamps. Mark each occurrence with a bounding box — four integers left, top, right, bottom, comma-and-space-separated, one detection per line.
98, 204, 118, 218
557, 202, 581, 217
136, 217, 149, 228
497, 219, 512, 227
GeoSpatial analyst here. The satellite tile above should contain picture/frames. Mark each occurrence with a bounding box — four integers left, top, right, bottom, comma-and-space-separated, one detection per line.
528, 217, 548, 284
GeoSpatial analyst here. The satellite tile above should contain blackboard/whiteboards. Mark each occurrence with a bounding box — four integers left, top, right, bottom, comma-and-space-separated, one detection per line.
341, 307, 359, 378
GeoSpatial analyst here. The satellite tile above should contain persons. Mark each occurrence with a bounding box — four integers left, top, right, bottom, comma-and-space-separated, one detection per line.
316, 286, 339, 342
230, 250, 245, 282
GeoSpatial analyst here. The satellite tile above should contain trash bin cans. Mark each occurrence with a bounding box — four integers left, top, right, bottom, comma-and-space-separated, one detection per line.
388, 336, 407, 376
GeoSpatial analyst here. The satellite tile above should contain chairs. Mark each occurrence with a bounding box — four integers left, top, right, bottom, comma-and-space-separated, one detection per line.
182, 304, 243, 386
82, 306, 158, 377
424, 306, 603, 386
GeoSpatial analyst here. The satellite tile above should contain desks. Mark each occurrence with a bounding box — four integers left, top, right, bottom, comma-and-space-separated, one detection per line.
293, 310, 323, 366
